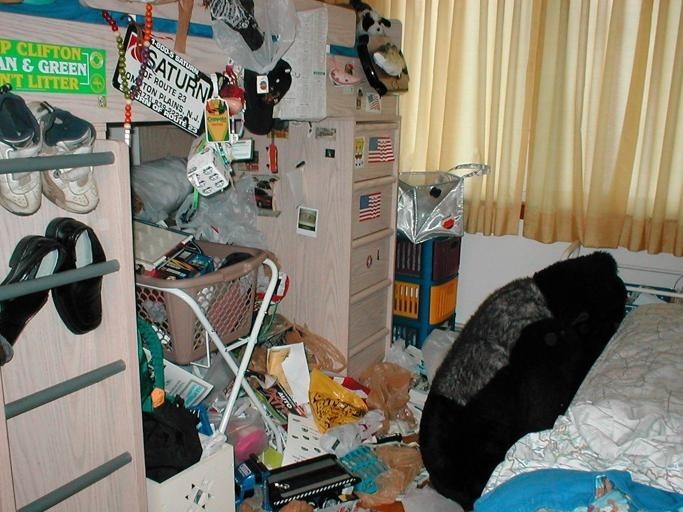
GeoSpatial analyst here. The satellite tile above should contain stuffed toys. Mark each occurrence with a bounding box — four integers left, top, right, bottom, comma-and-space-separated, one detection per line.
419, 251, 628, 510
349, 0, 391, 35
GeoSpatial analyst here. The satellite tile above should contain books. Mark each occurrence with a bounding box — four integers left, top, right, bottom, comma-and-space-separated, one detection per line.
141, 347, 215, 412
242, 342, 342, 468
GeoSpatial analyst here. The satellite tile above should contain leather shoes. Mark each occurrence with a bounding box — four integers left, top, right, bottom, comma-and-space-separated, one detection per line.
0, 234, 61, 361
45, 217, 108, 334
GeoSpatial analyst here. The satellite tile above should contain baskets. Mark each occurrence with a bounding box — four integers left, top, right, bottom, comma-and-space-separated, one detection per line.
392, 313, 456, 350
397, 238, 461, 280
133, 235, 268, 363
394, 276, 459, 325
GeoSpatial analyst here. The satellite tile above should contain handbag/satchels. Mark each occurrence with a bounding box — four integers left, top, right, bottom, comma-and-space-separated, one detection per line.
397, 163, 491, 241
141, 395, 203, 483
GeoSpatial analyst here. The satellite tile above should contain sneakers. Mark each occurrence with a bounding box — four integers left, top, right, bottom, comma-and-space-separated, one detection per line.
24, 99, 100, 214
0, 87, 43, 215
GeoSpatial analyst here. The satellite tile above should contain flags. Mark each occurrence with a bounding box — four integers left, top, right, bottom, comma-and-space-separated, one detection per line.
359, 191, 382, 221
367, 136, 395, 163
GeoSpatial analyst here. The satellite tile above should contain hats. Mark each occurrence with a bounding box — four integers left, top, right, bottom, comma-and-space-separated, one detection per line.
243, 56, 293, 136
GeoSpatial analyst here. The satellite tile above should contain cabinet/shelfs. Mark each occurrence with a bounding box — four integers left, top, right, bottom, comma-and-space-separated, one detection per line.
0, 1, 407, 512
394, 230, 462, 352
